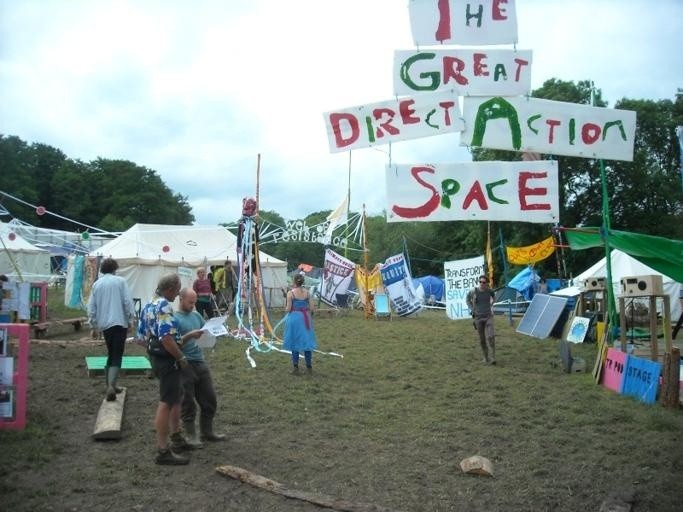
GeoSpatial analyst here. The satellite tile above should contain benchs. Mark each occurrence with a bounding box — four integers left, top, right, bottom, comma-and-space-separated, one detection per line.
29, 314, 87, 338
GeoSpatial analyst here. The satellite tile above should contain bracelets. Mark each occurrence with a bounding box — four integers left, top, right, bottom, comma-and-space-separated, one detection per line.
176, 355, 185, 362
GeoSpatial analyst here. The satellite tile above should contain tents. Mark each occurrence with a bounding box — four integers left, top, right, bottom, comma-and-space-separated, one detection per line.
62, 223, 290, 321
0, 220, 51, 286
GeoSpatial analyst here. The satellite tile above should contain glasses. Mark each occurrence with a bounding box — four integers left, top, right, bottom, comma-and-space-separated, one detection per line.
479, 280, 486, 284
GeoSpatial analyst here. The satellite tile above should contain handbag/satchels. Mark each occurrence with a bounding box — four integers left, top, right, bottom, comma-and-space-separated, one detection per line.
147, 336, 180, 358
199, 296, 210, 303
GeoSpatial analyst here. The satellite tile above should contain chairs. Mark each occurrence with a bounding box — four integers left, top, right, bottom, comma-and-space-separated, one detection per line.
131, 296, 143, 330
373, 292, 393, 323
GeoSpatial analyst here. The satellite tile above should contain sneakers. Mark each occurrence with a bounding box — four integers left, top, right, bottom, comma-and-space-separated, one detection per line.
172, 438, 205, 453
483, 354, 496, 364
154, 448, 189, 465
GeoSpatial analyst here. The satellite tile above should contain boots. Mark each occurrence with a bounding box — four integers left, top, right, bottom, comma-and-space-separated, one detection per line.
183, 416, 204, 449
302, 367, 312, 374
104, 366, 123, 401
198, 414, 226, 442
288, 367, 299, 375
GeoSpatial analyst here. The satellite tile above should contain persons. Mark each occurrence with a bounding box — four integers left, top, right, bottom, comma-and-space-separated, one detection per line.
0, 275, 10, 309
537, 278, 548, 294
192, 266, 214, 319
298, 267, 306, 276
135, 272, 204, 466
86, 259, 135, 401
212, 259, 237, 316
172, 286, 226, 448
466, 275, 499, 366
279, 274, 319, 374
